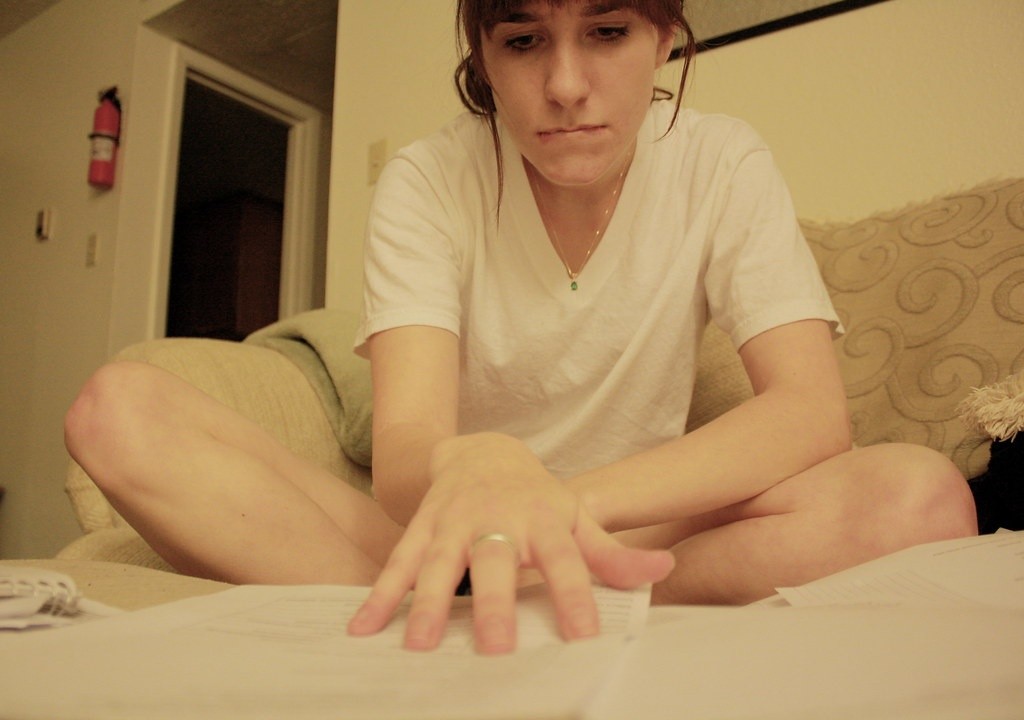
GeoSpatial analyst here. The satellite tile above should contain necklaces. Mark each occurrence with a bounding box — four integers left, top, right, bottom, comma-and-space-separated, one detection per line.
526, 148, 633, 290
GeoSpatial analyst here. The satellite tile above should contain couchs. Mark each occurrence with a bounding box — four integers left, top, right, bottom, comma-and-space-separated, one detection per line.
54, 336, 369, 574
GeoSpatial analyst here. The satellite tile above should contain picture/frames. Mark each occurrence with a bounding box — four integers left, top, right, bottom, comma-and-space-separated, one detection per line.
665, 0, 886, 63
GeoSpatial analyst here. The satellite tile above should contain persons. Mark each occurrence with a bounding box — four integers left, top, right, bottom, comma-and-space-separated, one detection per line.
65, 1, 979, 655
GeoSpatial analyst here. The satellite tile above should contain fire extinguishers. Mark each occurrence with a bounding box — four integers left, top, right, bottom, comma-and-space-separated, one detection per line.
88, 86, 122, 191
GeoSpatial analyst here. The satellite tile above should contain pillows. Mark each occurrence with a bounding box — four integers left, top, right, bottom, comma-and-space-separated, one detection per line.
686, 177, 1024, 478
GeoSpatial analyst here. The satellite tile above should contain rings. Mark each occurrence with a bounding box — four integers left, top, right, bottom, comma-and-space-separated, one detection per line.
468, 533, 521, 569
